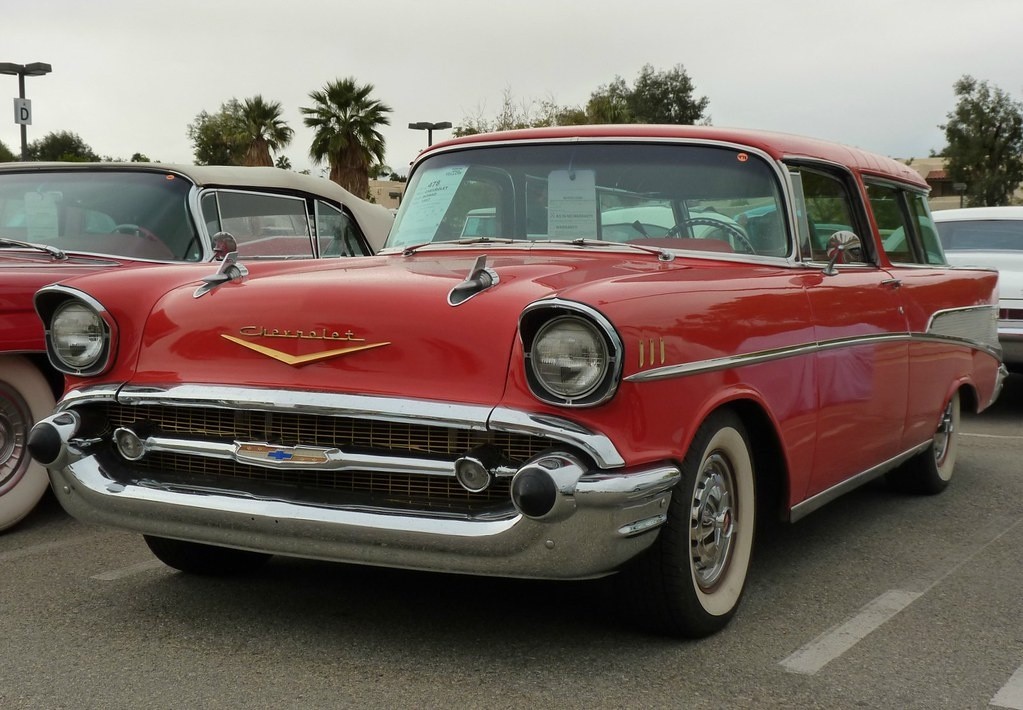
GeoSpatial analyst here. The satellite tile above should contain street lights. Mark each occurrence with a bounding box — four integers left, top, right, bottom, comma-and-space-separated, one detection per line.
408, 121, 452, 146
0, 61, 52, 161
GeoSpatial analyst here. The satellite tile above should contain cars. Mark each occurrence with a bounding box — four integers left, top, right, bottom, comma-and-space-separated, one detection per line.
880, 206, 1023, 364
0, 158, 394, 534
599, 206, 749, 252
24, 123, 1007, 641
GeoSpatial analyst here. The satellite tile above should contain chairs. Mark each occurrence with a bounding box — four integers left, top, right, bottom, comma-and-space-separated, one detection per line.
755, 209, 826, 254
